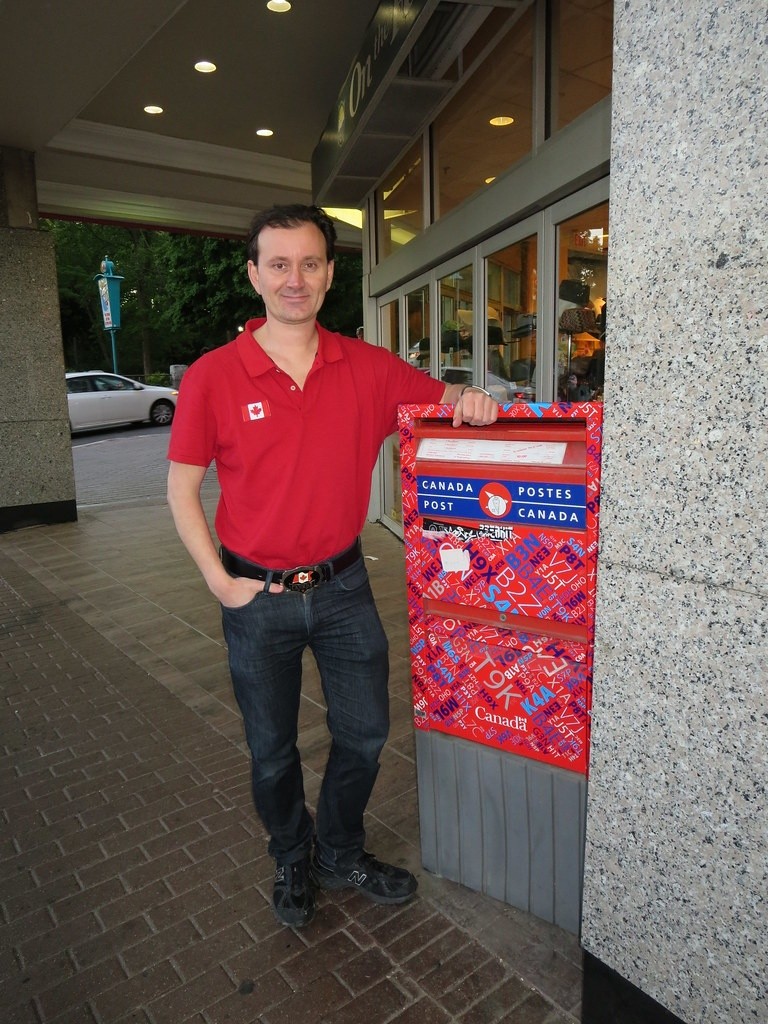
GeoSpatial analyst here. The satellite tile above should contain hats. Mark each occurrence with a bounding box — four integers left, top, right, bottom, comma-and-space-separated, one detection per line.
487, 307, 499, 320
441, 331, 467, 353
507, 314, 533, 331
509, 358, 536, 382
441, 368, 474, 384
416, 337, 430, 360
584, 303, 606, 342
558, 308, 602, 334
488, 326, 509, 346
559, 279, 595, 308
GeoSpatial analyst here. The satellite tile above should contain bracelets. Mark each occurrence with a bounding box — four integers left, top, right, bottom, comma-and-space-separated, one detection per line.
461, 385, 492, 398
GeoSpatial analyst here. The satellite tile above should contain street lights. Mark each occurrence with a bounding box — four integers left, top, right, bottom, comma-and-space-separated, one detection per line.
92, 254, 123, 375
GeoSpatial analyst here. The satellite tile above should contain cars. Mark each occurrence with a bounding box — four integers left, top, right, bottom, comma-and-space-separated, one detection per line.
389, 339, 472, 368
66, 370, 180, 439
426, 364, 536, 406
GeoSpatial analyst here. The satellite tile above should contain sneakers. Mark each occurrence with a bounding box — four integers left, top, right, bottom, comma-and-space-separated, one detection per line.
309, 835, 418, 905
272, 857, 313, 928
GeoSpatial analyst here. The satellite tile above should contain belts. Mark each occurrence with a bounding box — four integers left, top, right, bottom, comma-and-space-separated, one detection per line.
218, 536, 363, 592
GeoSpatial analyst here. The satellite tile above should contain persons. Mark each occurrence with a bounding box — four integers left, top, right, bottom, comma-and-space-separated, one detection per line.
165, 202, 497, 927
356, 327, 363, 339
